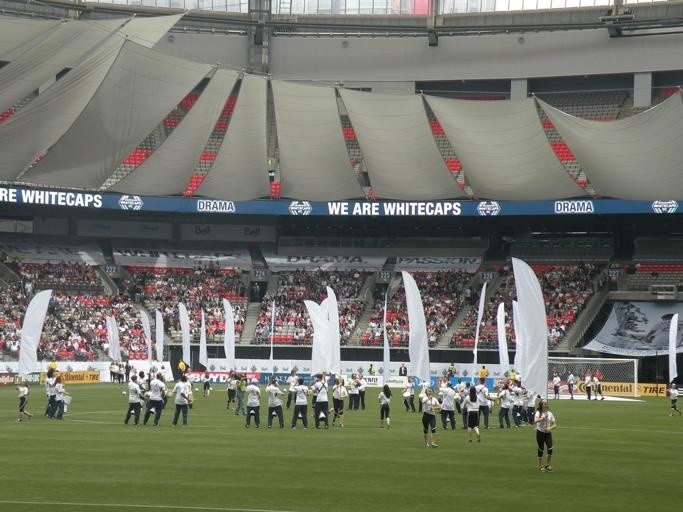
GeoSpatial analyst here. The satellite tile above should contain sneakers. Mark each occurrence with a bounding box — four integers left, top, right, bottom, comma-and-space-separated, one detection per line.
429, 442, 440, 448
124, 409, 191, 429
545, 465, 554, 473
538, 466, 546, 474
224, 403, 367, 430
376, 407, 537, 443
15, 411, 65, 423
552, 397, 605, 401
424, 442, 431, 448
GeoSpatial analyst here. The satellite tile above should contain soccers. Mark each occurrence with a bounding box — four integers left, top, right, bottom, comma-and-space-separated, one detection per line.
195, 387, 199, 392
209, 387, 213, 391
283, 388, 287, 393
122, 391, 126, 396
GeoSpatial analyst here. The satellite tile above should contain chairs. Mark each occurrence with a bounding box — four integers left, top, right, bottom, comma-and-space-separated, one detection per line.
100, 82, 240, 198
2, 88, 40, 121
0, 257, 608, 360
534, 86, 681, 121
623, 260, 683, 291
422, 95, 474, 198
18, 149, 48, 183
541, 121, 596, 197
333, 88, 373, 198
267, 82, 282, 198
